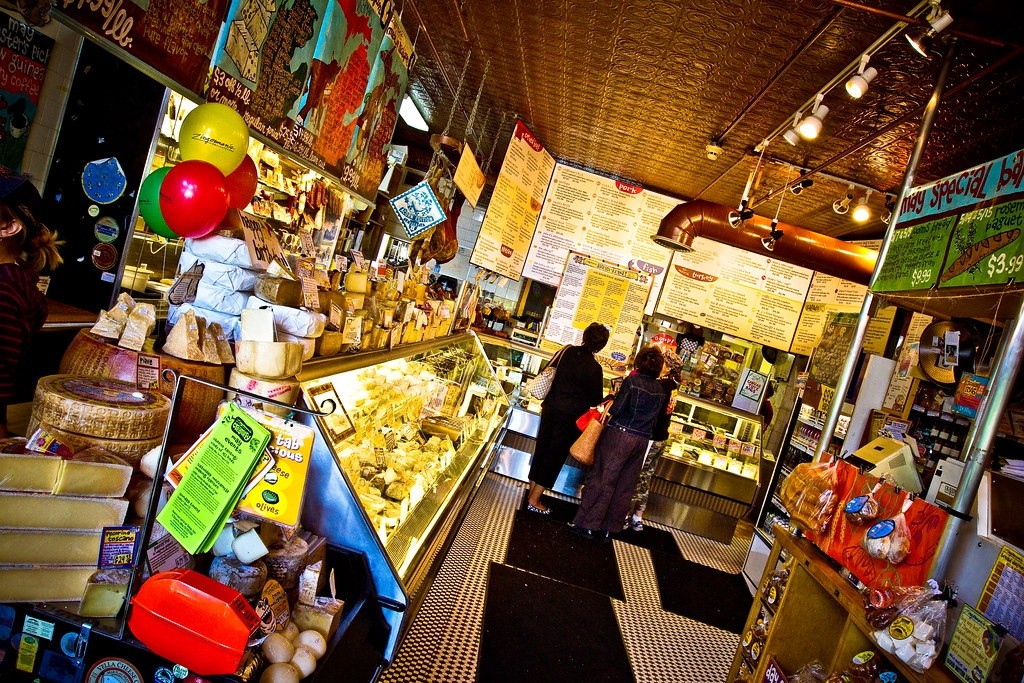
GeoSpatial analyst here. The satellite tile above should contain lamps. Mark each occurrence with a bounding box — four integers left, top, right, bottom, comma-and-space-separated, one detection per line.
845, 54, 878, 98
906, 0, 953, 57
880, 195, 896, 224
833, 183, 855, 214
761, 163, 794, 251
852, 189, 873, 224
728, 146, 766, 228
399, 93, 429, 132
790, 179, 813, 196
783, 112, 803, 146
799, 94, 829, 140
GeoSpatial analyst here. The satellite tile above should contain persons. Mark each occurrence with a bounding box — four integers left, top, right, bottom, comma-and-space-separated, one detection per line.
0, 172, 65, 433
946, 345, 957, 363
567, 347, 666, 543
526, 322, 610, 516
623, 351, 684, 534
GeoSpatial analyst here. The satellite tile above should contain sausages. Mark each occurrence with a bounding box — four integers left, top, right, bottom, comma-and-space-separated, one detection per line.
306, 182, 341, 218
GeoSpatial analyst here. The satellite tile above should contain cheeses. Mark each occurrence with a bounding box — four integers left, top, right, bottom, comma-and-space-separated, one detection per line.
331, 356, 473, 538
0, 435, 345, 643
87, 272, 454, 419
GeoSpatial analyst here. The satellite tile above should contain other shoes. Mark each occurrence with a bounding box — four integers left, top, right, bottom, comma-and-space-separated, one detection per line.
526, 499, 551, 515
623, 519, 628, 530
628, 518, 644, 531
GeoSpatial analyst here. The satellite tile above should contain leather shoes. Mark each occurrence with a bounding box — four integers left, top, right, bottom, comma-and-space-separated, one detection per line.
598, 529, 610, 542
566, 521, 594, 539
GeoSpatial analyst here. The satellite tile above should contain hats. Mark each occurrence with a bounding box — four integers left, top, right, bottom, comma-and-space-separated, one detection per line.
0, 174, 43, 223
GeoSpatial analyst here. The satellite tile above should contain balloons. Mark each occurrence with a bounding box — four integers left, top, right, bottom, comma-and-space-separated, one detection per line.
179, 103, 250, 179
138, 167, 180, 240
159, 160, 231, 239
223, 155, 258, 212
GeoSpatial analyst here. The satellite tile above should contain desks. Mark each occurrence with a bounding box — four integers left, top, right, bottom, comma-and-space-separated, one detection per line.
0, 602, 392, 683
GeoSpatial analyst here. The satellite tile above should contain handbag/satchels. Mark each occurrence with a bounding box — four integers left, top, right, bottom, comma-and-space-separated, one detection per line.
779, 455, 840, 535
827, 472, 974, 589
869, 579, 958, 674
803, 451, 880, 567
526, 344, 573, 400
570, 400, 614, 466
576, 399, 611, 432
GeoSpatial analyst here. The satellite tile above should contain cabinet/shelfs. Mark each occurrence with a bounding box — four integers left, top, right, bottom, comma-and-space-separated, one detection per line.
728, 524, 955, 683
43, 38, 376, 314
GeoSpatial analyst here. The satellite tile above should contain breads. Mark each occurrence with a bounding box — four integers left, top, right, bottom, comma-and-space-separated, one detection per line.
862, 521, 911, 564
780, 463, 838, 532
845, 498, 878, 525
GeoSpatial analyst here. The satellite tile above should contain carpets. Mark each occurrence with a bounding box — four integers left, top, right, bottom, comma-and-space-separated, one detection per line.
474, 489, 763, 683
